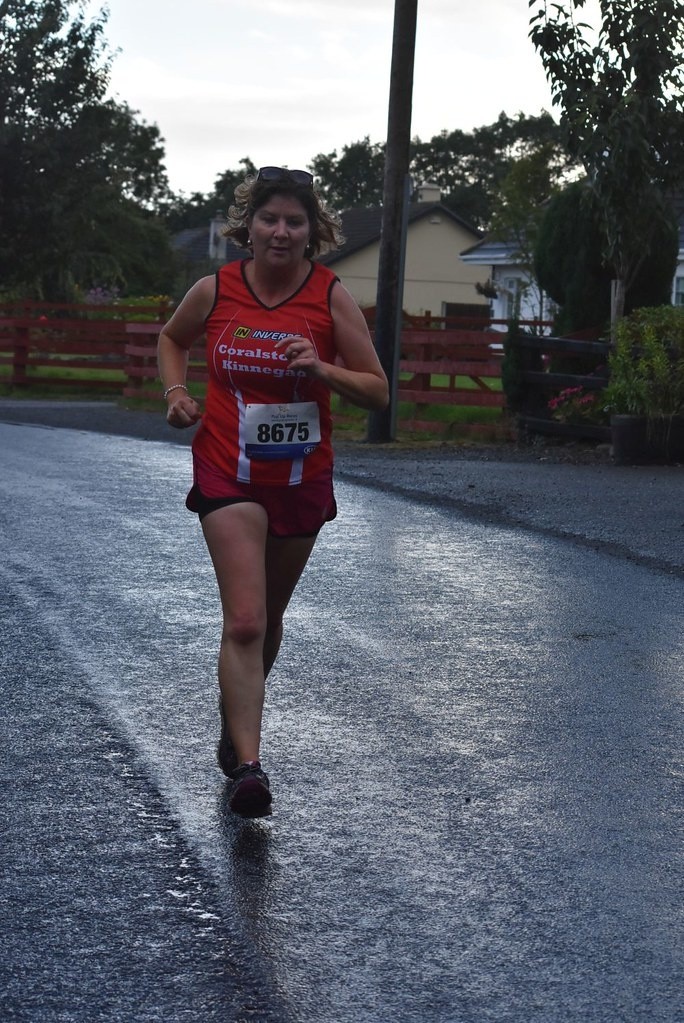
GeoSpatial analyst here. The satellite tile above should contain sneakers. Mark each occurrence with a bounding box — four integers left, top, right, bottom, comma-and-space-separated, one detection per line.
229, 759, 274, 819
217, 693, 238, 779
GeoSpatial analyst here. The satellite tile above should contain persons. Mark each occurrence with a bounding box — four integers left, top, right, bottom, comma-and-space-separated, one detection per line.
156, 168, 390, 817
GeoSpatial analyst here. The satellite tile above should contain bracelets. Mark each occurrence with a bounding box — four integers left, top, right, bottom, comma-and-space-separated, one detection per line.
163, 385, 188, 400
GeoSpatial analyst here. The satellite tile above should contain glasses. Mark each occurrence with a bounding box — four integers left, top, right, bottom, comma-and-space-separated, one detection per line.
256, 166, 313, 191
